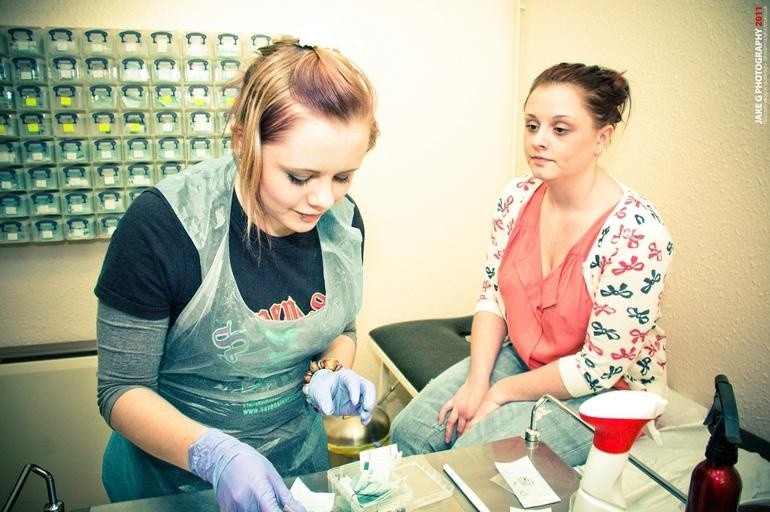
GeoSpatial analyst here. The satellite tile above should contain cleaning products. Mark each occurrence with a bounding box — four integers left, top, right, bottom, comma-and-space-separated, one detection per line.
680, 373, 745, 512
565, 389, 671, 512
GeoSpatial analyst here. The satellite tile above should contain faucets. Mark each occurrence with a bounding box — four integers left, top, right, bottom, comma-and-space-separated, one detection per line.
2, 461, 69, 512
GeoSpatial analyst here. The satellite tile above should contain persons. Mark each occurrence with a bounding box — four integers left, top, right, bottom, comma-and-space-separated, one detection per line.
92, 34, 384, 512
387, 62, 677, 470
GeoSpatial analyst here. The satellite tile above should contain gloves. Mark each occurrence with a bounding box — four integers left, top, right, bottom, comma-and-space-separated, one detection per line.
302, 366, 377, 428
187, 428, 307, 512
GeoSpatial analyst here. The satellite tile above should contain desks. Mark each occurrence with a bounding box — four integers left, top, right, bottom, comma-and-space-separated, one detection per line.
86, 436, 585, 511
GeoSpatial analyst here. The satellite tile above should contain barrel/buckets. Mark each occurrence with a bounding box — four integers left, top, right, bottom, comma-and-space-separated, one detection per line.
323, 403, 392, 469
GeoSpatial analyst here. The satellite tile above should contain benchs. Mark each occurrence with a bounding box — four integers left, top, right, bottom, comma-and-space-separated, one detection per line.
367, 314, 770, 511
